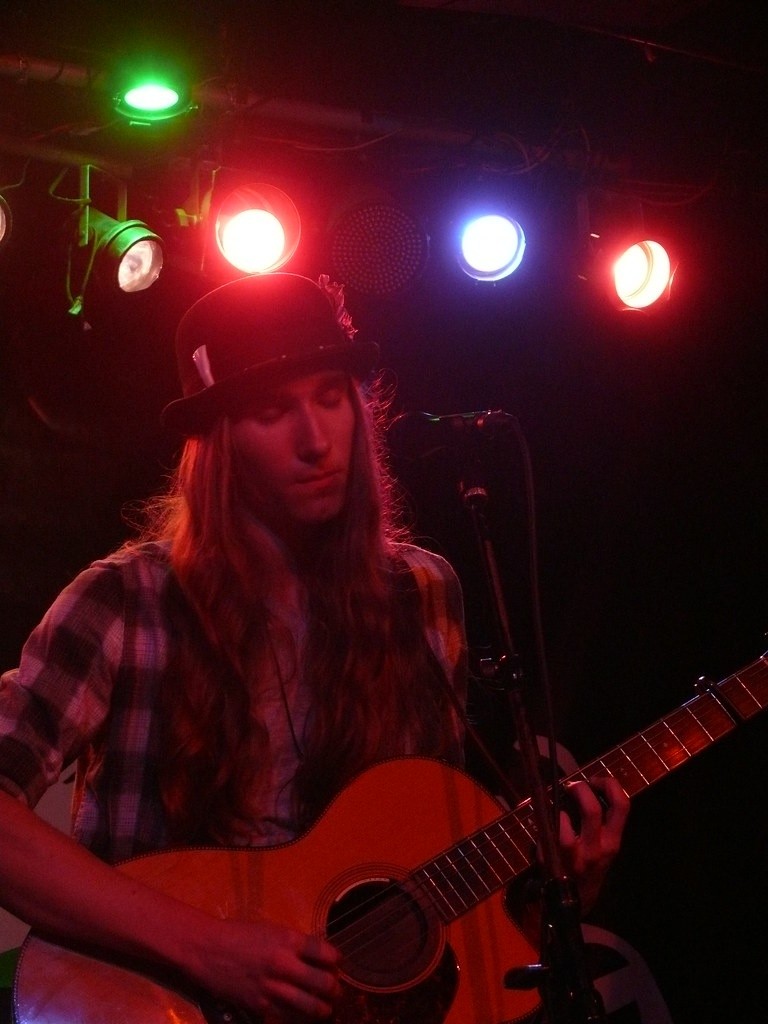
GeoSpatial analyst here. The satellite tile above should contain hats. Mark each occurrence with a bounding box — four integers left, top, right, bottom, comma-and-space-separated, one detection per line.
159, 272, 355, 425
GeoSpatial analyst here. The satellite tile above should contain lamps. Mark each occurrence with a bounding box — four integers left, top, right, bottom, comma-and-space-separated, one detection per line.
573, 216, 692, 316
328, 185, 429, 300
199, 168, 312, 280
62, 204, 166, 292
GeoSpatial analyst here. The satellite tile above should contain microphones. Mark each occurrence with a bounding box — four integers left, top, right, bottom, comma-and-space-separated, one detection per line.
385, 409, 513, 459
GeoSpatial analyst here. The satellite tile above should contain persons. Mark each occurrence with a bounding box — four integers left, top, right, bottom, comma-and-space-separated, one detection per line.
0, 273, 628, 1024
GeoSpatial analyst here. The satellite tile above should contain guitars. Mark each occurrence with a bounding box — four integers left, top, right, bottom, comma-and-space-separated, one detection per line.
11, 650, 768, 1024
